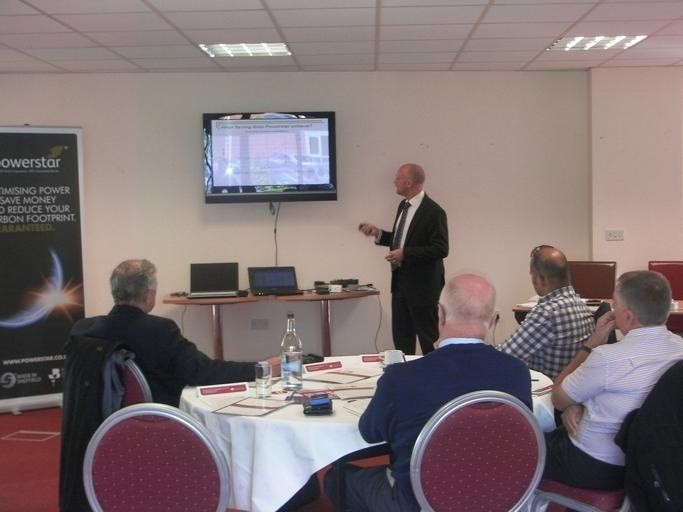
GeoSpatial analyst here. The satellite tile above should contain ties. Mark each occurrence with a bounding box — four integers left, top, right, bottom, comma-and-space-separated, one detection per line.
390, 202, 410, 271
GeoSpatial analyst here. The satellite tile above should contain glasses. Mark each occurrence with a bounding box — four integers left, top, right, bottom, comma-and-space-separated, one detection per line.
531, 245, 554, 281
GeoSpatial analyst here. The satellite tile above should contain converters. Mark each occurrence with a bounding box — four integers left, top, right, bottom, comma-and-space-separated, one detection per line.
316, 285, 329, 294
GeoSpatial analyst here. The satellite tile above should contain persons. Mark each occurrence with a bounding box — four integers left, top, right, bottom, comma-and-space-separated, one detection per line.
358, 161, 450, 356
322, 273, 534, 511
67, 257, 284, 406
492, 244, 598, 435
541, 270, 682, 493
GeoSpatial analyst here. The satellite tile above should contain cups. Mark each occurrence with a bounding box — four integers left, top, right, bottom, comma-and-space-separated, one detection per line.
254, 361, 272, 396
328, 284, 342, 293
380, 350, 403, 364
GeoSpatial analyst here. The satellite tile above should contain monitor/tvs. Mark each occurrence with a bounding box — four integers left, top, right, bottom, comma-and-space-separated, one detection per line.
202, 111, 337, 204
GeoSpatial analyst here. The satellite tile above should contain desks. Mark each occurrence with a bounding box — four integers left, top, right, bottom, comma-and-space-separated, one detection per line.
178, 353, 554, 512
512, 295, 683, 331
163, 289, 380, 361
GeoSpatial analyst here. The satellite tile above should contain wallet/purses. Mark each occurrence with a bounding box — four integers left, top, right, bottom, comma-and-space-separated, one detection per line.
303, 398, 332, 416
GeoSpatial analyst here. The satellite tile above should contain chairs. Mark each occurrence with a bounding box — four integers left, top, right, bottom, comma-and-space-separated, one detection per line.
567, 260, 683, 299
82, 360, 232, 512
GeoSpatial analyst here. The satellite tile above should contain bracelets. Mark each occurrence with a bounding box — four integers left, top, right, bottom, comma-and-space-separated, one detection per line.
578, 344, 592, 357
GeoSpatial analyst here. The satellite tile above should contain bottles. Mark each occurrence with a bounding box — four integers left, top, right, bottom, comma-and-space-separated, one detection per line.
281, 311, 303, 393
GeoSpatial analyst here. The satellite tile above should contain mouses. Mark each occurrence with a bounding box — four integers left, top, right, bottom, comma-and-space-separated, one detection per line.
239, 290, 247, 297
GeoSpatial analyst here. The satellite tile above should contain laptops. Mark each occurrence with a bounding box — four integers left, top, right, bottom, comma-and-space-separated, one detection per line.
189, 262, 239, 298
247, 267, 304, 296
